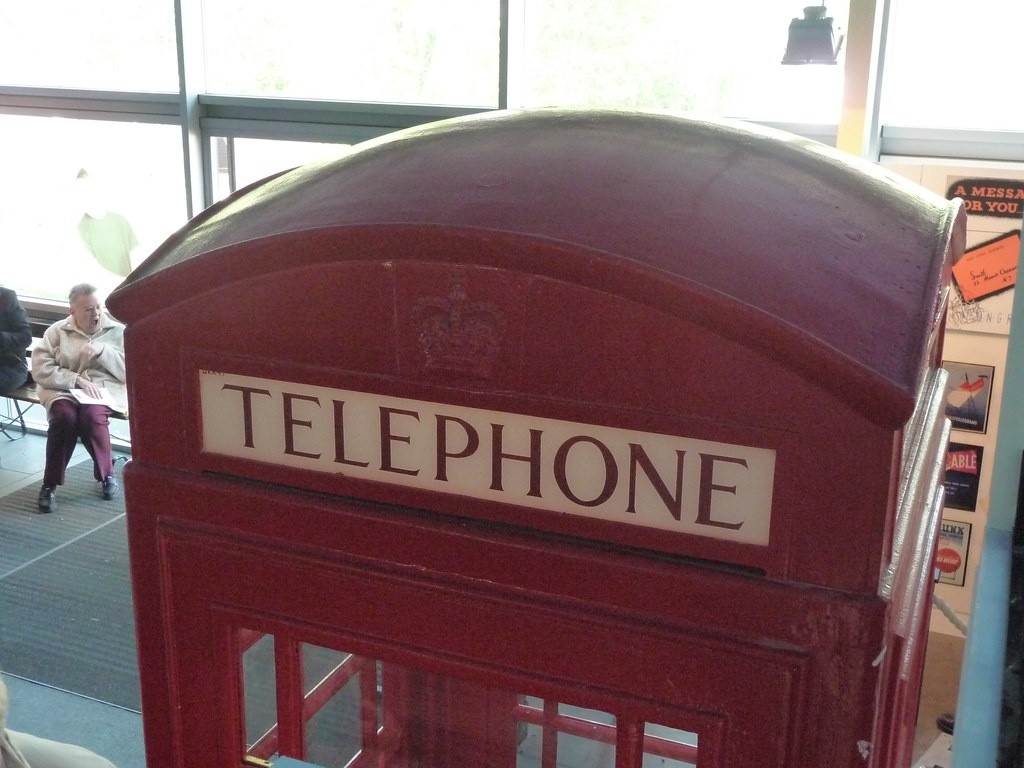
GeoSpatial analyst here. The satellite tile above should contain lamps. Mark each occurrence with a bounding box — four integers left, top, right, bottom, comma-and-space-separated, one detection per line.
781, 2, 844, 66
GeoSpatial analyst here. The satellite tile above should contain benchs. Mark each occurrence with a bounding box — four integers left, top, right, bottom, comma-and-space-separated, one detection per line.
0, 321, 129, 468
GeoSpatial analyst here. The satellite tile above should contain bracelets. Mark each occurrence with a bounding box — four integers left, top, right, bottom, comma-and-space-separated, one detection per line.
77, 378, 84, 385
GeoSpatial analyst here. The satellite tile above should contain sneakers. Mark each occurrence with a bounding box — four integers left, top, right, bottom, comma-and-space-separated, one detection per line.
38, 485, 56, 513
102, 476, 121, 500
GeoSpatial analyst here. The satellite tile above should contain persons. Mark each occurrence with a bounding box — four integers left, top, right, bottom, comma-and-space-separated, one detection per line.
0, 287, 33, 398
30, 283, 129, 513
1, 681, 121, 768
936, 712, 955, 733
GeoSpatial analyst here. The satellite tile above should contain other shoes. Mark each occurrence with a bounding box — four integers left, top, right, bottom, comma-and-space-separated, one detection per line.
934, 712, 955, 736
